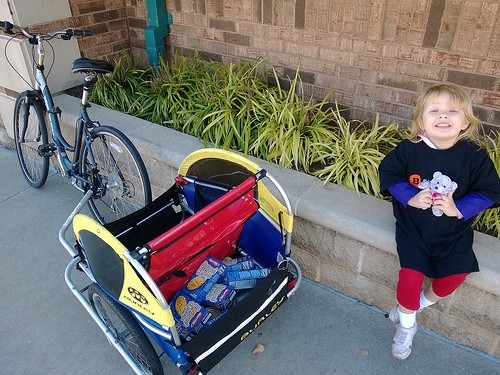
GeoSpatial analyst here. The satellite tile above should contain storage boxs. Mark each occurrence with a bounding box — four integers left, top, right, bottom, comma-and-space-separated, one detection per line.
168, 255, 272, 342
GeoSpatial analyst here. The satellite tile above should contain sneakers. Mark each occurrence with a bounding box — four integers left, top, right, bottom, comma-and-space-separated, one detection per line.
389, 321, 419, 362
388, 288, 425, 323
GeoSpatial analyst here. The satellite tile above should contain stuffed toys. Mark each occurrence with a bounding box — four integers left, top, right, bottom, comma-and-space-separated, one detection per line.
417, 171, 459, 217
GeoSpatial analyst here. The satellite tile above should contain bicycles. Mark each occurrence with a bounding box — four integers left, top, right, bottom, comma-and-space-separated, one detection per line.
0, 21, 151, 231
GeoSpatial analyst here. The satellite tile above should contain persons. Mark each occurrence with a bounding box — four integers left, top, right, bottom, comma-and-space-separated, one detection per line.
378, 83, 500, 358
